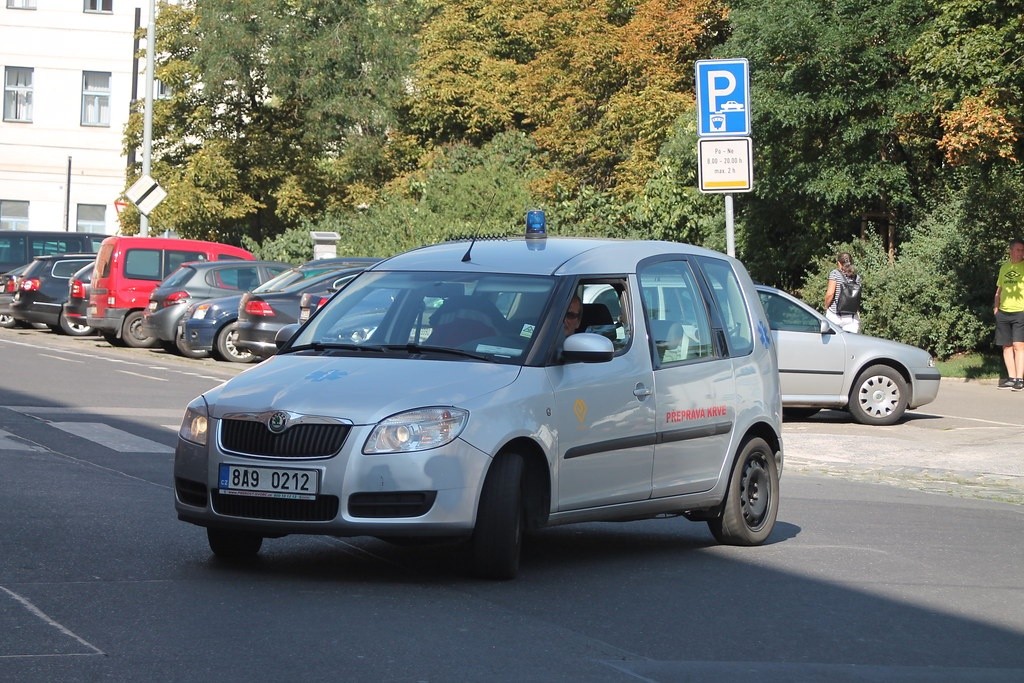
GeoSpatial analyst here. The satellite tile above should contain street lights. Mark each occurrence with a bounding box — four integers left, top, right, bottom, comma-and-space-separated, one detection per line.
65, 156, 72, 232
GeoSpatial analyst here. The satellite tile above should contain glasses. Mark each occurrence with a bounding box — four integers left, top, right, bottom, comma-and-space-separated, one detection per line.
565, 312, 580, 321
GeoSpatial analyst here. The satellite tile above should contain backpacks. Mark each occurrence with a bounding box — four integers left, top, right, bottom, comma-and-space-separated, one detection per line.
837, 283, 859, 315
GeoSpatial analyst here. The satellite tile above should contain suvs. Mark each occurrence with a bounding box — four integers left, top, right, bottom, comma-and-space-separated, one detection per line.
491, 274, 941, 426
9, 252, 97, 336
0, 242, 66, 265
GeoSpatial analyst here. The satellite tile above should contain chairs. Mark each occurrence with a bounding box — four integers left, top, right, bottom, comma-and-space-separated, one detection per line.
424, 296, 499, 346
576, 303, 617, 341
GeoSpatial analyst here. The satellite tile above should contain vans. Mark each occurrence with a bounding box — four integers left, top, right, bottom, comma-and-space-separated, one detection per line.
0, 230, 115, 328
86, 236, 276, 348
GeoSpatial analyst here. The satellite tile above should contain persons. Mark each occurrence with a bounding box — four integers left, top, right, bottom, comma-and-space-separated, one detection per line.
564, 295, 582, 335
993, 240, 1024, 392
824, 253, 861, 334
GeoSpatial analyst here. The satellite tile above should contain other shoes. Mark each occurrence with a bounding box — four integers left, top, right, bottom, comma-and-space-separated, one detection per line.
996, 377, 1024, 392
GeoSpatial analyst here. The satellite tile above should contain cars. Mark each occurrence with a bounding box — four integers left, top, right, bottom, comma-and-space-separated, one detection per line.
231, 257, 388, 356
141, 260, 298, 358
0, 263, 31, 316
297, 283, 440, 324
175, 211, 783, 580
62, 261, 95, 325
177, 294, 258, 364
